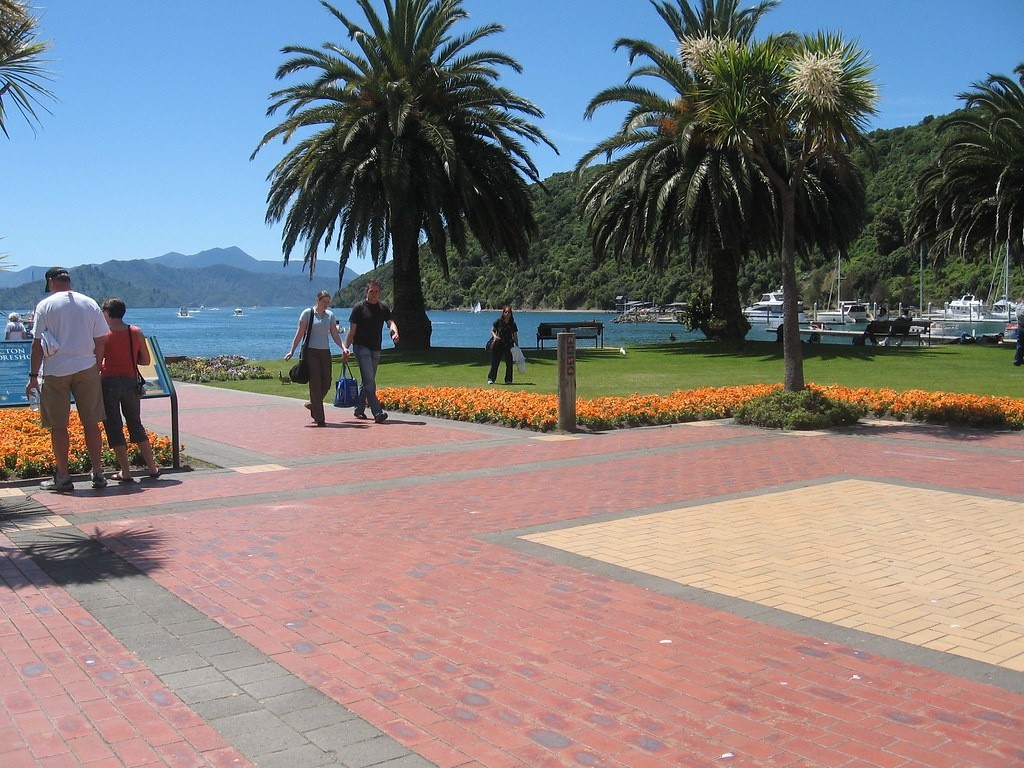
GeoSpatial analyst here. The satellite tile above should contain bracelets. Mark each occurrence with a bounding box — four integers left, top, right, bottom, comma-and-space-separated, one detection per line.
29, 373, 38, 377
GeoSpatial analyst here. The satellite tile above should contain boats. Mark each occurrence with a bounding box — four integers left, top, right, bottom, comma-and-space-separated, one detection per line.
234, 308, 242, 315
180, 306, 189, 316
336, 325, 346, 333
817, 303, 868, 323
741, 286, 808, 328
949, 293, 983, 317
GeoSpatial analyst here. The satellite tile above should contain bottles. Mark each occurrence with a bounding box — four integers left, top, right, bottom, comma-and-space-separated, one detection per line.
28, 388, 39, 411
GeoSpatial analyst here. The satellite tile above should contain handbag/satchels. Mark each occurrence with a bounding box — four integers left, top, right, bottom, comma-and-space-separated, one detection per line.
334, 362, 358, 407
485, 337, 496, 350
289, 363, 309, 384
137, 382, 146, 396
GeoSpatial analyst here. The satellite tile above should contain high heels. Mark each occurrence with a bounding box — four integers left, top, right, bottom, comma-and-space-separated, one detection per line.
304, 403, 312, 410
315, 419, 325, 426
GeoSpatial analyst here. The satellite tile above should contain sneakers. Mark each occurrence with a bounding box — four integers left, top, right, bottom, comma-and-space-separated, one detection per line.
354, 412, 367, 419
507, 381, 511, 384
92, 472, 107, 487
40, 475, 74, 491
375, 413, 388, 423
488, 380, 493, 384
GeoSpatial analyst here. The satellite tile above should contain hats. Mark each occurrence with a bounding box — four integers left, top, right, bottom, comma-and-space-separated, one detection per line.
44, 267, 69, 293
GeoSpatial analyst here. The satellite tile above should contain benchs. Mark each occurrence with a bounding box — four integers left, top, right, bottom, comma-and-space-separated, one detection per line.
864, 321, 929, 352
536, 322, 605, 351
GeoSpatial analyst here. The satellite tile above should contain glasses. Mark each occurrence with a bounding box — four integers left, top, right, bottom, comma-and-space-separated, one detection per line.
505, 311, 510, 313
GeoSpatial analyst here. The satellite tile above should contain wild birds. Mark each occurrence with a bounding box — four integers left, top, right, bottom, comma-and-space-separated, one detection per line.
619, 347, 626, 356
669, 333, 677, 341
277, 371, 292, 384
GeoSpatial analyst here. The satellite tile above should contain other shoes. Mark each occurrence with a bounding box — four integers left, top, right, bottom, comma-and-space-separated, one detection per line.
879, 341, 885, 346
895, 342, 901, 345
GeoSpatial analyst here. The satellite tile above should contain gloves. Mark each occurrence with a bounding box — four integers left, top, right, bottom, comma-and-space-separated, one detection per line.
29, 373, 38, 376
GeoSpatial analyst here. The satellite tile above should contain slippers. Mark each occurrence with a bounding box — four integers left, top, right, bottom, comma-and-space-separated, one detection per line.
150, 467, 161, 477
111, 474, 133, 480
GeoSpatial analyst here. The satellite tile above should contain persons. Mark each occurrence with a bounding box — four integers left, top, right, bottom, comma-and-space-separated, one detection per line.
960, 332, 1004, 345
335, 320, 345, 333
24, 267, 112, 491
4, 312, 27, 341
879, 307, 913, 346
487, 306, 519, 384
344, 280, 399, 423
285, 291, 351, 427
670, 332, 676, 341
1013, 294, 1024, 366
95, 298, 161, 480
776, 323, 783, 343
852, 308, 889, 346
